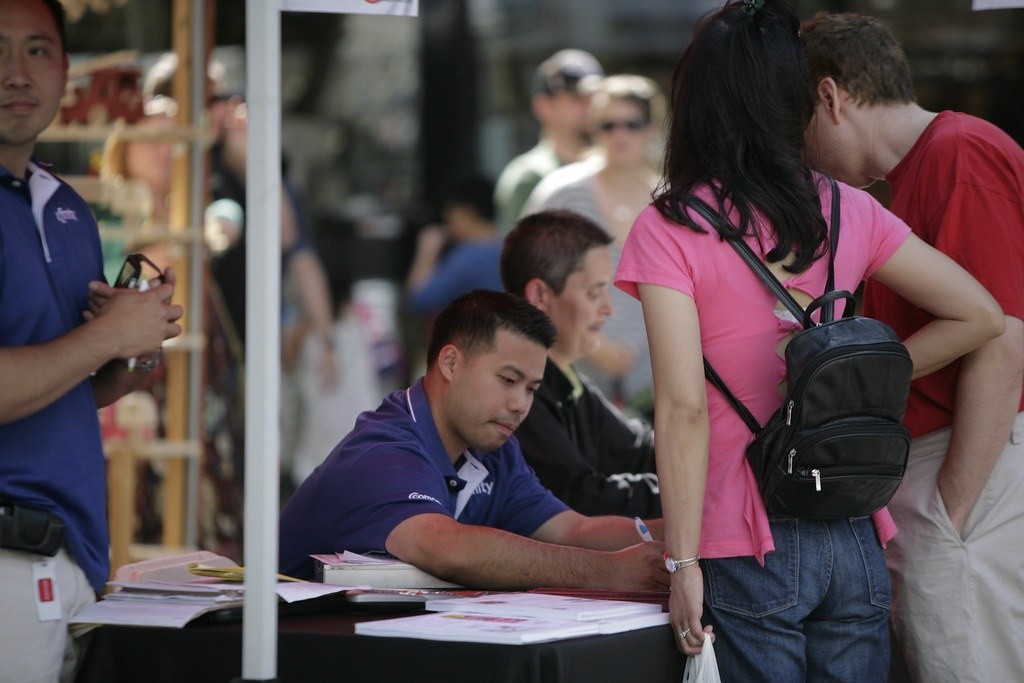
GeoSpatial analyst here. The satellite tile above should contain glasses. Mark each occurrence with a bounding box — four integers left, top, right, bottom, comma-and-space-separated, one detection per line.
115, 252, 163, 290
594, 118, 655, 134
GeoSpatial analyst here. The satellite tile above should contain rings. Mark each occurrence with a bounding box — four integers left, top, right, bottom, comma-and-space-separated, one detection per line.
680, 628, 690, 639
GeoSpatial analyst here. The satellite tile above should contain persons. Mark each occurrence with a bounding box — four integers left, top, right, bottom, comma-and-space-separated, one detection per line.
500, 208, 664, 520
0, 0, 183, 683
492, 48, 669, 415
105, 54, 339, 387
612, 0, 1005, 683
799, 12, 1024, 683
407, 170, 503, 313
276, 288, 674, 592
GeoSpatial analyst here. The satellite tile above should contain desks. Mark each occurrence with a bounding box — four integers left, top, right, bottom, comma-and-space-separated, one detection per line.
50, 592, 688, 683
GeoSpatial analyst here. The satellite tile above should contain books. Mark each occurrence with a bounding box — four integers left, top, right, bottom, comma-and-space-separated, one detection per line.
354, 591, 672, 646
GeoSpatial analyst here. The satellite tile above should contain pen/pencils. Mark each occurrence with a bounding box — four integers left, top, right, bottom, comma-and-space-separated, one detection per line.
635, 517, 653, 542
127, 275, 150, 373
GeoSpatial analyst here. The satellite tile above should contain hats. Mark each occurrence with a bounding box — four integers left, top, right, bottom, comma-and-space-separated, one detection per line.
527, 49, 605, 96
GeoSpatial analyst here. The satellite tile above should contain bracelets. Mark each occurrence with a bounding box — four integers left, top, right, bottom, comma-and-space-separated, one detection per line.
122, 358, 162, 370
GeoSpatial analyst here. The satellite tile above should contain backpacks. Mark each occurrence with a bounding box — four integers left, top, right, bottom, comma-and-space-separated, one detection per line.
685, 178, 913, 519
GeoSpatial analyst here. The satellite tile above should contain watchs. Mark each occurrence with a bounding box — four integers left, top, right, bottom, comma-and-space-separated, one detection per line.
664, 553, 700, 573
